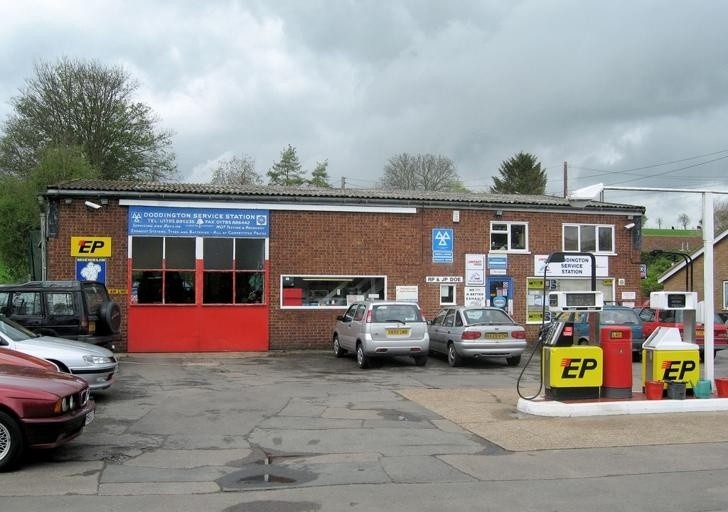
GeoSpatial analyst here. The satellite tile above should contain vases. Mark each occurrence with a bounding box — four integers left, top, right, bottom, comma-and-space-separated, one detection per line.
644, 378, 728, 399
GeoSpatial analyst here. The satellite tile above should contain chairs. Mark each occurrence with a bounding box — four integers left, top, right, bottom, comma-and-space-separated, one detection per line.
53, 302, 66, 311
376, 309, 401, 316
479, 316, 488, 322
601, 312, 626, 321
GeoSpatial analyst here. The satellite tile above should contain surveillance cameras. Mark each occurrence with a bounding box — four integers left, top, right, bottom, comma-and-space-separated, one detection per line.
85, 201, 103, 210
623, 222, 635, 230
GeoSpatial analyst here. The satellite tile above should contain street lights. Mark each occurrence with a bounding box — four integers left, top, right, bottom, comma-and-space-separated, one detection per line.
647, 249, 694, 291
563, 183, 727, 398
545, 250, 597, 291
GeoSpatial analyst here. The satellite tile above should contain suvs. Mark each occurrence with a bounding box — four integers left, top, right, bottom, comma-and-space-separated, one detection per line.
0, 278, 123, 348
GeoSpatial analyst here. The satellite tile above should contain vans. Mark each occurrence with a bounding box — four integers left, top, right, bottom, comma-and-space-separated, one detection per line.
0, 349, 97, 470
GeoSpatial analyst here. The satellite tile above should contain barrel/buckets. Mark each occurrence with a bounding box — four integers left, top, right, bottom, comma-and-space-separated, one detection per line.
645, 381, 663, 398
716, 379, 727, 397
695, 378, 711, 398
666, 381, 686, 399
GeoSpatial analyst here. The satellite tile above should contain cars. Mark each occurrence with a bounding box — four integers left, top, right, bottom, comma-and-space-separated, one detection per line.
331, 299, 431, 370
630, 304, 728, 363
0, 311, 119, 394
574, 304, 647, 361
429, 304, 529, 368
